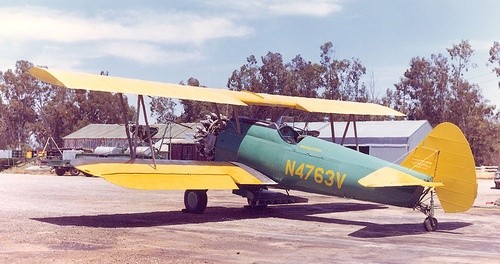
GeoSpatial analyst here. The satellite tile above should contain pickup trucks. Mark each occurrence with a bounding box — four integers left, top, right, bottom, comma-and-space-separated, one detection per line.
50, 151, 92, 176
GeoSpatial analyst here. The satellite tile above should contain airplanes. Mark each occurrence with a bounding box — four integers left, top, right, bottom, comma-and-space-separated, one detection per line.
29, 67, 479, 232
